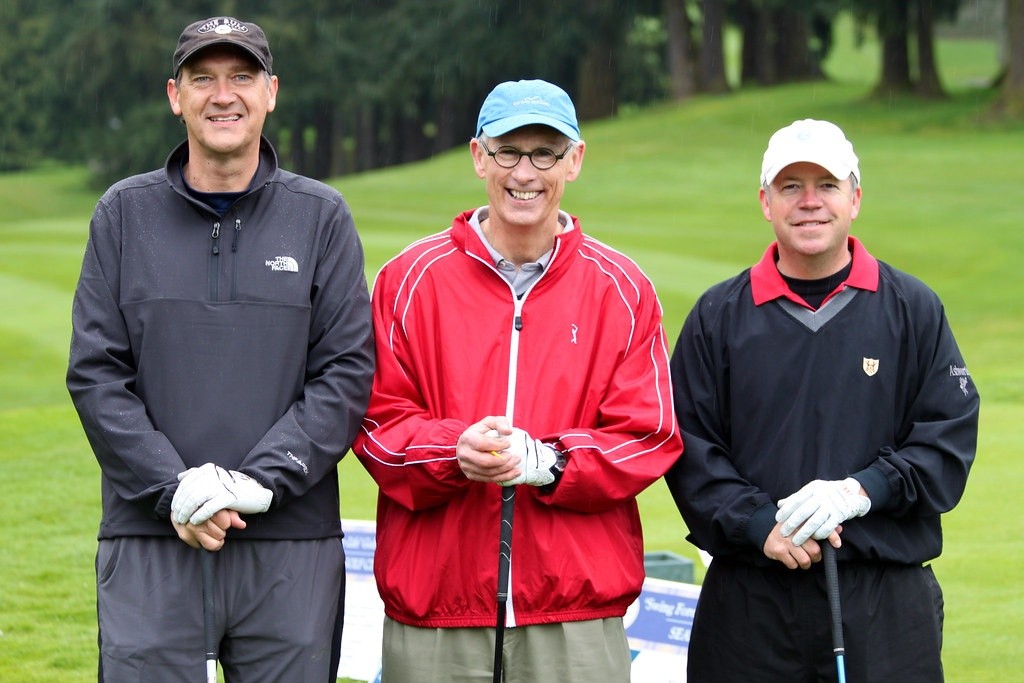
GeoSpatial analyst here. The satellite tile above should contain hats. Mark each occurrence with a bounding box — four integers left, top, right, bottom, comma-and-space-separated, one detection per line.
476, 79, 580, 142
760, 119, 859, 189
173, 17, 273, 76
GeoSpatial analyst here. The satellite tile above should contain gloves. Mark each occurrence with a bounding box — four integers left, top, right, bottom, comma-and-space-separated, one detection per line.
171, 463, 272, 525
776, 478, 870, 545
486, 428, 559, 491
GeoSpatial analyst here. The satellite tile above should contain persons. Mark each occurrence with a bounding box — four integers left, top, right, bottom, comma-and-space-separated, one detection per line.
663, 119, 980, 683
67, 15, 377, 682
348, 80, 684, 682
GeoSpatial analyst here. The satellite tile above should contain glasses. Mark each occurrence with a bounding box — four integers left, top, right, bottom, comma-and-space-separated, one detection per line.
480, 141, 572, 170
